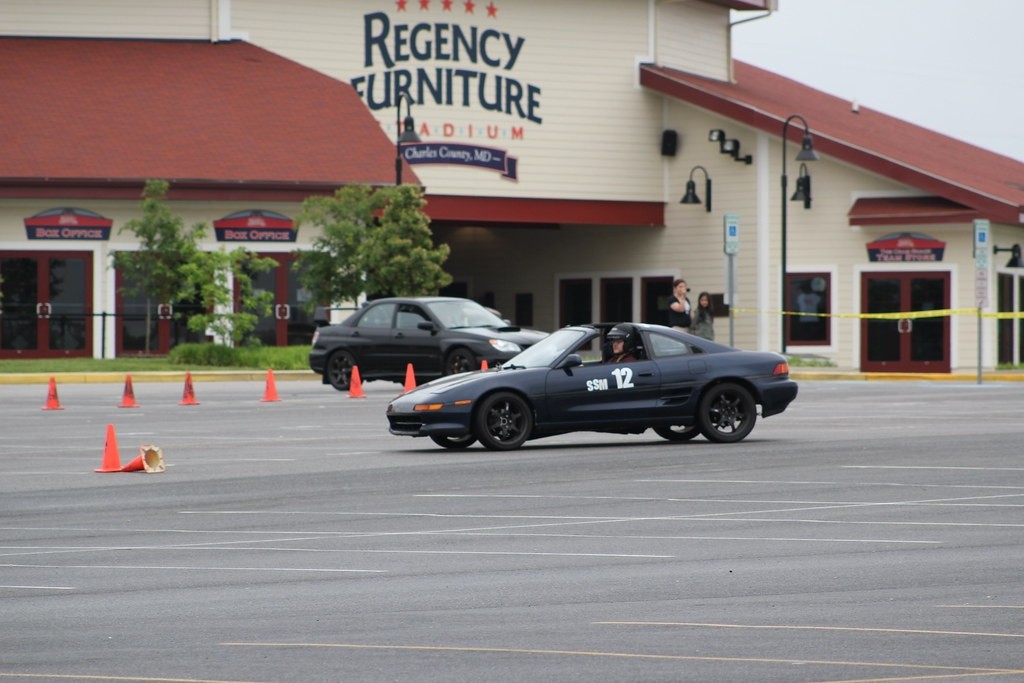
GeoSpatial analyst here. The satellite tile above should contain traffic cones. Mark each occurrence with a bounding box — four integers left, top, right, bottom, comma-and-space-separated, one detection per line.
122, 443, 167, 473
117, 374, 141, 408
481, 359, 489, 371
345, 365, 367, 399
41, 376, 65, 411
178, 368, 201, 405
94, 423, 124, 472
400, 364, 417, 395
260, 369, 283, 403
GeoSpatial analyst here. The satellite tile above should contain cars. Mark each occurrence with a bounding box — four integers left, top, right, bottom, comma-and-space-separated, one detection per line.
306, 296, 554, 391
386, 322, 798, 451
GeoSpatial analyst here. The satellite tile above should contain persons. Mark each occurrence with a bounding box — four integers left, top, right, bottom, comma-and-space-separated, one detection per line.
688, 291, 714, 341
665, 279, 693, 332
598, 323, 639, 365
794, 280, 826, 341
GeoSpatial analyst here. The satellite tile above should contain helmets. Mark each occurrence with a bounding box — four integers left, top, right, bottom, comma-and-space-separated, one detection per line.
606, 323, 638, 352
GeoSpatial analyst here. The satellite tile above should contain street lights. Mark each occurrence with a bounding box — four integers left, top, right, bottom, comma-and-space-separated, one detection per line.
393, 92, 423, 185
974, 242, 1024, 385
781, 109, 818, 354
